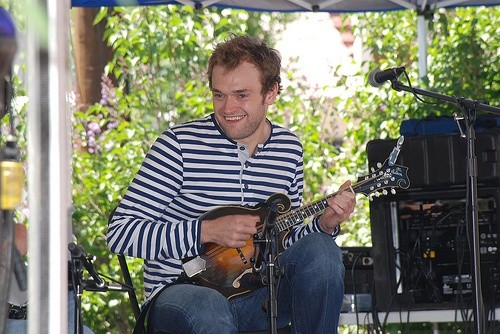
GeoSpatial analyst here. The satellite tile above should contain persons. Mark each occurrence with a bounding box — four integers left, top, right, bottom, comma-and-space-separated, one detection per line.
107, 35, 356, 334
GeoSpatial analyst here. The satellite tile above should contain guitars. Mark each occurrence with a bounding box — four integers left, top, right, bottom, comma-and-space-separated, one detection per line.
182, 135, 411, 301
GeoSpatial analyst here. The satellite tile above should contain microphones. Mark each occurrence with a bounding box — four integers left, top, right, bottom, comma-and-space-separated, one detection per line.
368, 68, 403, 87
241, 273, 269, 290
69, 279, 132, 293
